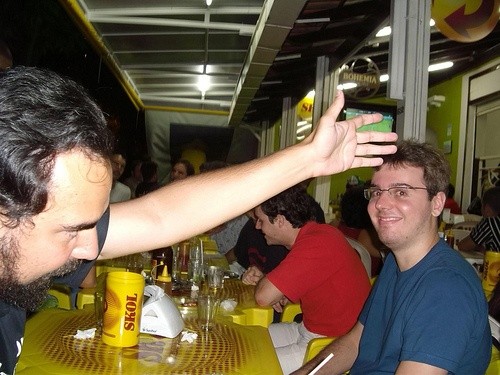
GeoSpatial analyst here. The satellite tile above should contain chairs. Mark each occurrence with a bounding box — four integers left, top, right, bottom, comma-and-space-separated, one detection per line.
282, 239, 381, 366
450, 220, 500, 375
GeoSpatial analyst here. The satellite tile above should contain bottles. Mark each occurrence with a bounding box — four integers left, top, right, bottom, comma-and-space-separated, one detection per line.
96, 253, 145, 325
179, 242, 189, 274
188, 236, 204, 280
151, 246, 173, 277
150, 264, 173, 296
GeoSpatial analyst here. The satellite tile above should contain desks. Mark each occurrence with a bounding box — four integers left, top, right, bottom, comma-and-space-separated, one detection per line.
15, 234, 284, 375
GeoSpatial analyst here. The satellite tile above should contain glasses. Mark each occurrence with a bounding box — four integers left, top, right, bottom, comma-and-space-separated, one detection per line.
363, 187, 435, 201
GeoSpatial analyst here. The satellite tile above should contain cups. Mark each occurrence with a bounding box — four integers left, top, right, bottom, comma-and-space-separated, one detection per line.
100, 271, 146, 347
197, 292, 220, 332
208, 265, 223, 288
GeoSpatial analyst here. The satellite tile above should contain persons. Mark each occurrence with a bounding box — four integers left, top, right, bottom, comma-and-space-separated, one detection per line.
0, 64, 398, 375
199, 162, 372, 375
135, 162, 166, 200
338, 185, 387, 279
111, 149, 131, 203
291, 138, 493, 375
445, 180, 462, 215
170, 160, 195, 182
458, 188, 500, 350
